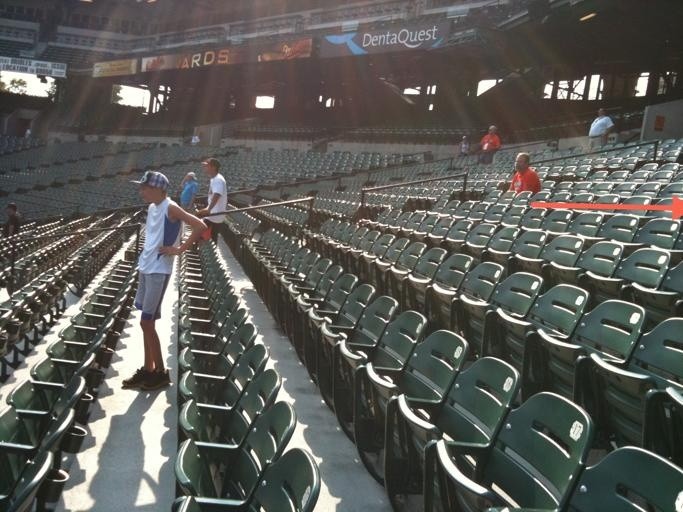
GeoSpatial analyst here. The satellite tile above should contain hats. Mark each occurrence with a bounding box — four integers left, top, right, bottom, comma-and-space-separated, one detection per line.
187, 172, 198, 181
129, 171, 169, 190
203, 158, 221, 167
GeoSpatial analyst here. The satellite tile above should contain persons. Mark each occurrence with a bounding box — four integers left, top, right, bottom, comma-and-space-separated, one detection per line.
508, 152, 541, 194
459, 136, 470, 157
588, 109, 614, 148
473, 125, 501, 164
122, 171, 208, 390
181, 171, 199, 214
24, 126, 31, 140
3, 203, 21, 237
198, 159, 228, 228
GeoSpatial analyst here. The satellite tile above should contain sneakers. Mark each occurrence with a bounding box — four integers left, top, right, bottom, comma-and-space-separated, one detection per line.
122, 368, 154, 387
141, 369, 171, 389
